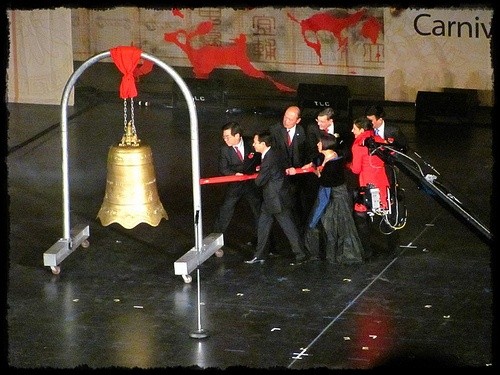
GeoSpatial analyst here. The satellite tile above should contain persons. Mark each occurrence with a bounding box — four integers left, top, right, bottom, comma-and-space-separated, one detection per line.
244, 130, 308, 266
304, 134, 366, 263
212, 121, 279, 257
247, 106, 312, 248
304, 106, 358, 199
364, 105, 409, 260
344, 117, 396, 266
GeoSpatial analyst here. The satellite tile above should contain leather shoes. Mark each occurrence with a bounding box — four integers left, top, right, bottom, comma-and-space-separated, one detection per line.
288, 258, 309, 267
267, 246, 279, 256
243, 255, 265, 265
246, 236, 258, 246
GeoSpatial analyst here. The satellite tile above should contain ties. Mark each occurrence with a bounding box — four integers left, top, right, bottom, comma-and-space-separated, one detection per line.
323, 128, 329, 136
234, 147, 243, 163
374, 129, 380, 135
287, 130, 292, 147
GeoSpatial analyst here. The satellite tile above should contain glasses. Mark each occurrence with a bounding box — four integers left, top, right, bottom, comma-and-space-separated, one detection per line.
316, 119, 325, 123
222, 136, 230, 140
